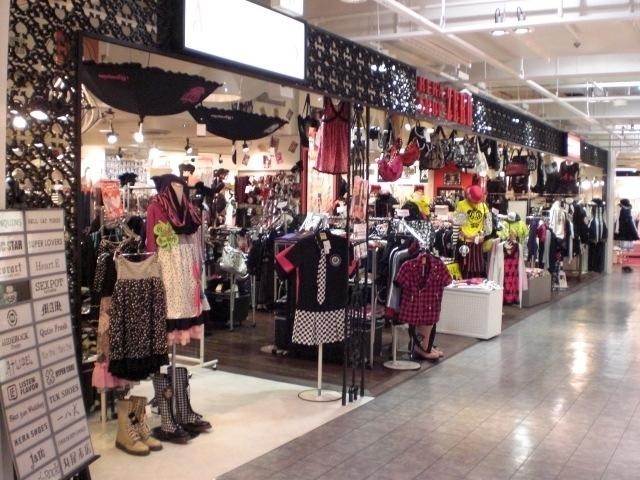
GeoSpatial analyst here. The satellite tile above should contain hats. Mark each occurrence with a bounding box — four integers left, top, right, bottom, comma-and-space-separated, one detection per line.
467, 185, 483, 203
618, 199, 632, 209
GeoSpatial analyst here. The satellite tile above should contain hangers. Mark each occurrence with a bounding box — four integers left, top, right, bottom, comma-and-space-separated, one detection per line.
261, 210, 436, 252
113, 237, 159, 259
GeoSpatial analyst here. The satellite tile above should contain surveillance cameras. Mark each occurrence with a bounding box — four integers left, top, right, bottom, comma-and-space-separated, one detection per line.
573, 40, 581, 47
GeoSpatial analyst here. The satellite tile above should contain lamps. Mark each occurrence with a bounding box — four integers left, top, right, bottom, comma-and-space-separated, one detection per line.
107, 119, 118, 144
130, 112, 150, 144
184, 135, 195, 156
491, 9, 508, 36
201, 70, 240, 104
515, 8, 530, 34
30, 99, 50, 123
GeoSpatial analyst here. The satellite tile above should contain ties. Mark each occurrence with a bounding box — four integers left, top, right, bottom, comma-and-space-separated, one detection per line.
317, 248, 326, 306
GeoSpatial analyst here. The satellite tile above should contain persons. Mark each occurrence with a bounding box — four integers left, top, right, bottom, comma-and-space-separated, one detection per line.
452, 185, 492, 285
414, 328, 444, 360
619, 199, 640, 273
119, 172, 138, 212
147, 182, 211, 345
549, 201, 567, 273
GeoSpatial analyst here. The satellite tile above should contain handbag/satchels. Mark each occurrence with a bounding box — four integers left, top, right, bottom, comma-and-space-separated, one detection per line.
219, 232, 247, 275
398, 121, 489, 178
482, 203, 493, 237
505, 148, 536, 176
377, 146, 403, 181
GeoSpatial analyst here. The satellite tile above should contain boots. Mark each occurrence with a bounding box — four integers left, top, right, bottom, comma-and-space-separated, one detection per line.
129, 395, 163, 450
167, 366, 212, 431
116, 399, 150, 455
153, 376, 191, 443
413, 324, 440, 360
